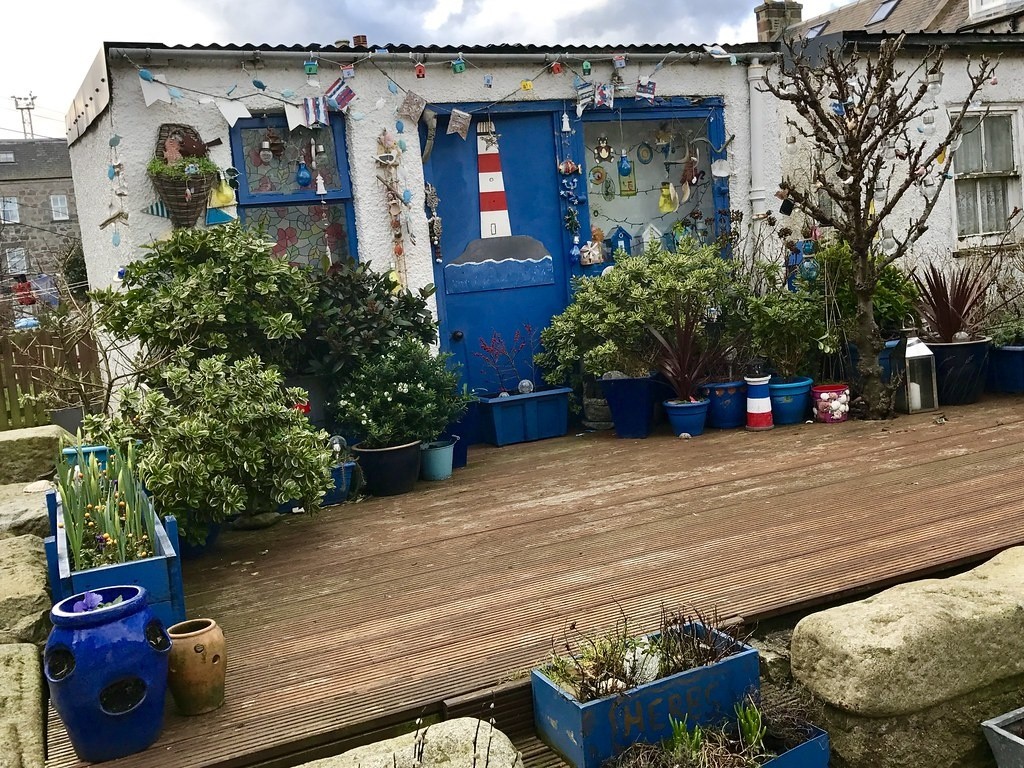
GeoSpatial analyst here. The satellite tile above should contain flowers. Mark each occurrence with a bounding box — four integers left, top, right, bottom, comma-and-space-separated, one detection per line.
325, 336, 482, 445
72, 590, 122, 614
473, 321, 543, 393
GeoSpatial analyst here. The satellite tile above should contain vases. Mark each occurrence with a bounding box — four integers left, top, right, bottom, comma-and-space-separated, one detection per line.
169, 619, 232, 716
351, 439, 423, 496
422, 435, 461, 482
45, 585, 173, 765
478, 386, 574, 446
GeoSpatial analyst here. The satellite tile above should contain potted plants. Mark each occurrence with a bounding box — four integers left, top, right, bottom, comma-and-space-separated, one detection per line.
18, 152, 1024, 768
